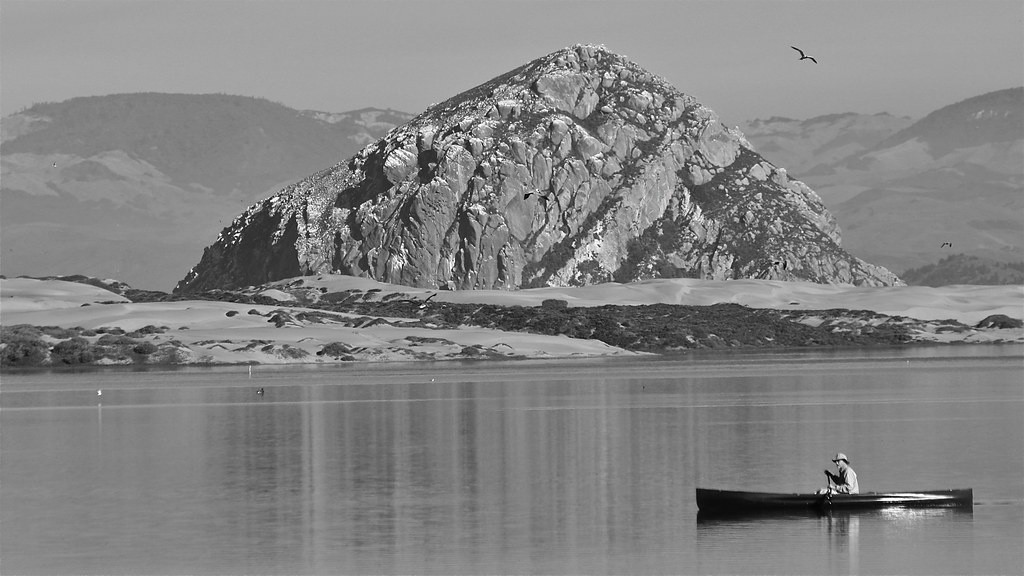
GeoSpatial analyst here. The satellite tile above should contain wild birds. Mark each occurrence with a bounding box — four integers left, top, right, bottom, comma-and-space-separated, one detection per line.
790, 45, 818, 64
642, 386, 646, 389
97, 389, 103, 396
940, 242, 953, 249
429, 378, 435, 382
256, 388, 265, 394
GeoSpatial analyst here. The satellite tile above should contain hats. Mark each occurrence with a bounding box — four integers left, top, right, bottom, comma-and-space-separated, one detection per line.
831, 453, 849, 464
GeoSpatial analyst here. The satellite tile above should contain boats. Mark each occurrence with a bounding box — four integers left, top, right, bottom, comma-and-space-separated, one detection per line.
695, 485, 974, 512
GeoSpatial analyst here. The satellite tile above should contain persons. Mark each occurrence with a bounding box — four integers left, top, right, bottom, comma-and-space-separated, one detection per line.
824, 453, 859, 494
